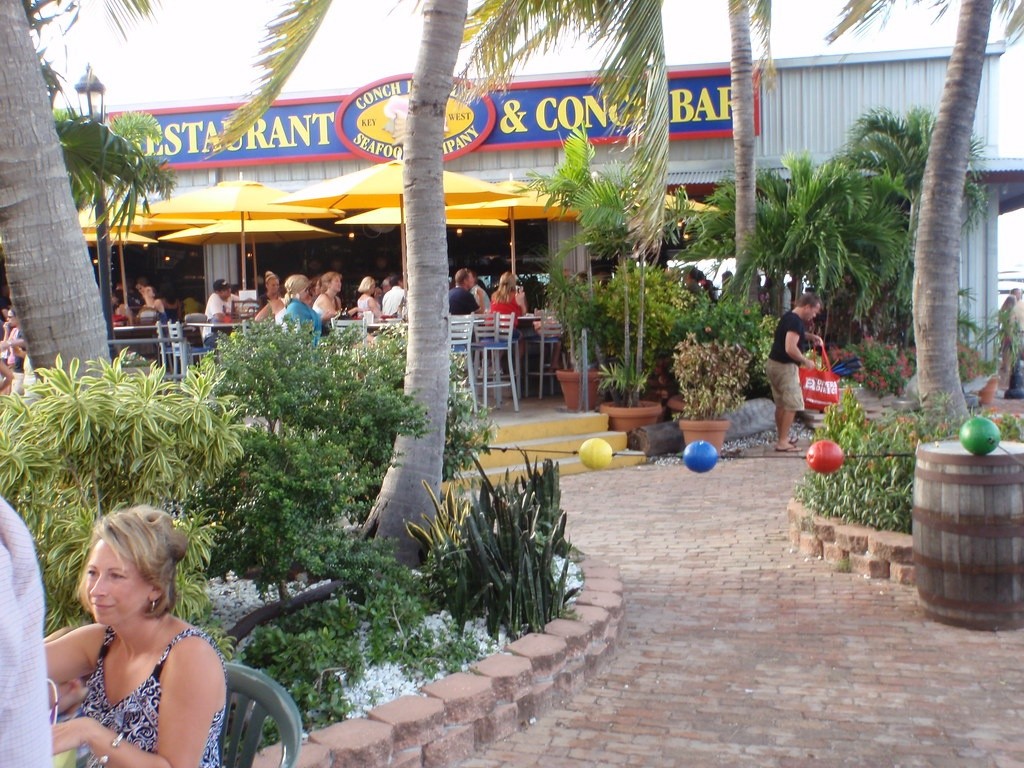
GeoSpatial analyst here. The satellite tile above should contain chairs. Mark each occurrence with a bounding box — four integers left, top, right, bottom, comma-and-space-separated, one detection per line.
157, 312, 559, 413
220, 664, 303, 768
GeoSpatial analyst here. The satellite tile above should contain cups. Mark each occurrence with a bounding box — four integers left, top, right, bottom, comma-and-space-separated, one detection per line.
364, 310, 374, 324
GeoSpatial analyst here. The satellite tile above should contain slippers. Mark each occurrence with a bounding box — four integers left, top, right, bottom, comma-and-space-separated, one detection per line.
788, 437, 799, 446
774, 444, 803, 453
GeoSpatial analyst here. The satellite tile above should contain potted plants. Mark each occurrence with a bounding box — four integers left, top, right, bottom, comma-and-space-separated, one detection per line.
555, 254, 779, 458
978, 311, 1024, 406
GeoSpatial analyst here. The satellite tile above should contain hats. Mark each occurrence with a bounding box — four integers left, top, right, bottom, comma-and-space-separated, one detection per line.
212, 278, 230, 290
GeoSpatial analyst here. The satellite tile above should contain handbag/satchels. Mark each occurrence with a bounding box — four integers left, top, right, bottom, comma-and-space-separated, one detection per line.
798, 340, 841, 411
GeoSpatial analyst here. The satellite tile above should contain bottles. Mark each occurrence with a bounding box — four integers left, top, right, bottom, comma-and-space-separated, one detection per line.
402, 303, 408, 322
397, 305, 401, 318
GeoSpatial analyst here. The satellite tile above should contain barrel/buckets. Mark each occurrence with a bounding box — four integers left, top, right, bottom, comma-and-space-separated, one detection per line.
912, 442, 1024, 629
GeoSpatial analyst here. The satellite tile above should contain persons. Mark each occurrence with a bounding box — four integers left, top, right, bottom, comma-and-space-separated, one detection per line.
1000, 289, 1024, 374
0, 307, 29, 399
0, 497, 53, 768
769, 292, 824, 452
112, 273, 256, 344
681, 265, 803, 318
39, 505, 227, 768
255, 271, 579, 403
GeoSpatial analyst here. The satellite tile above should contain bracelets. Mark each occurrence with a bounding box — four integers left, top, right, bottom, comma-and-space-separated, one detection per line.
99, 732, 128, 764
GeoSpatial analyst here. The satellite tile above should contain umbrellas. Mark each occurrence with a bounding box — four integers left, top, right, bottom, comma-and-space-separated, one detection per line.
76, 160, 729, 328
